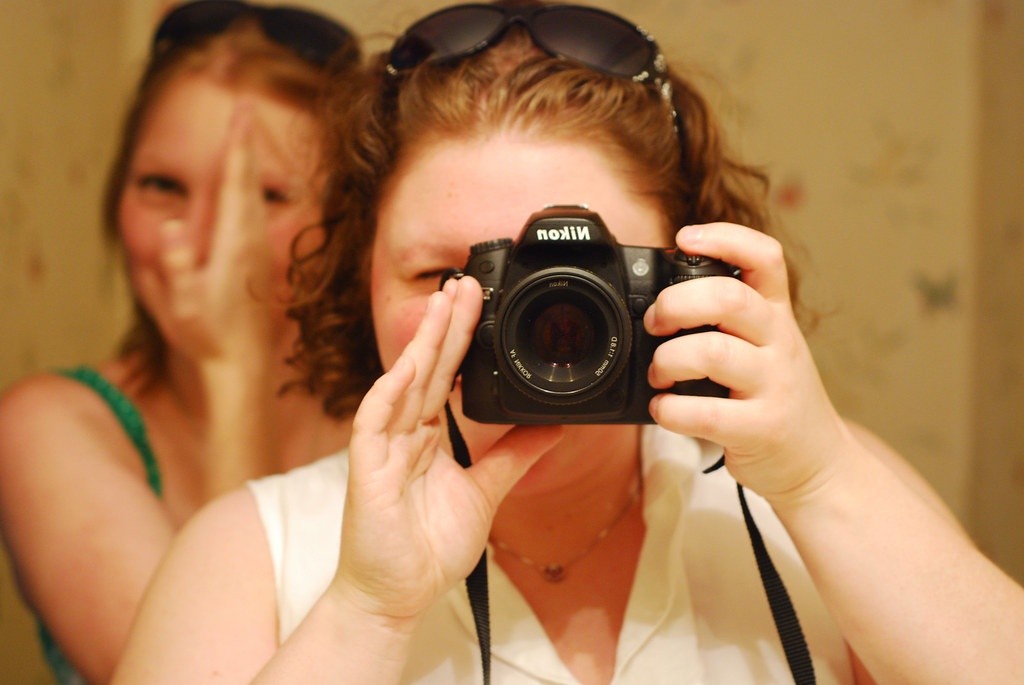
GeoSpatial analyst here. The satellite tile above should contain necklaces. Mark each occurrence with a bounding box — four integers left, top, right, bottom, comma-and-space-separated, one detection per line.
488, 486, 631, 582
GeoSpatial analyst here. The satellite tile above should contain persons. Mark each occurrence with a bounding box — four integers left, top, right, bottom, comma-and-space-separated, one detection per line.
112, 1, 1024, 685
1, 0, 358, 685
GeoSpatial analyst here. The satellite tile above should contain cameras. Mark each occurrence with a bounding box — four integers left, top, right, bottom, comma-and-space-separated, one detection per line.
451, 205, 741, 428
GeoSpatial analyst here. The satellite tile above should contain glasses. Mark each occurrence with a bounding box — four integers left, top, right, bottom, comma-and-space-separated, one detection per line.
382, 4, 688, 165
153, 0, 361, 78
